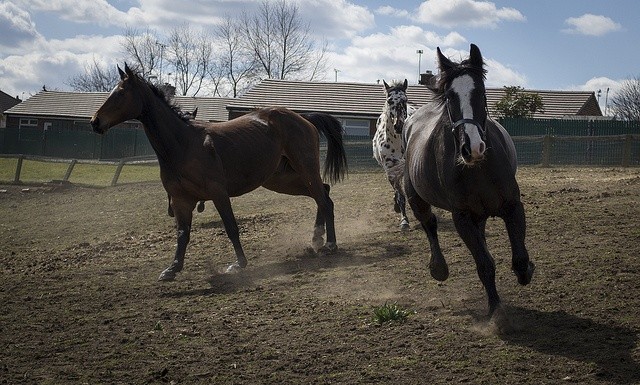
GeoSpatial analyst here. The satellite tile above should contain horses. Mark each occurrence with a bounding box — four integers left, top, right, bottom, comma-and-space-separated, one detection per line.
373, 79, 422, 230
88, 62, 350, 280
401, 42, 536, 322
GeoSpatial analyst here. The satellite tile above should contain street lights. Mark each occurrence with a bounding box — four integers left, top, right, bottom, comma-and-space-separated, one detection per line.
334, 67, 341, 81
155, 40, 167, 83
605, 86, 610, 115
416, 48, 424, 83
596, 89, 602, 105
